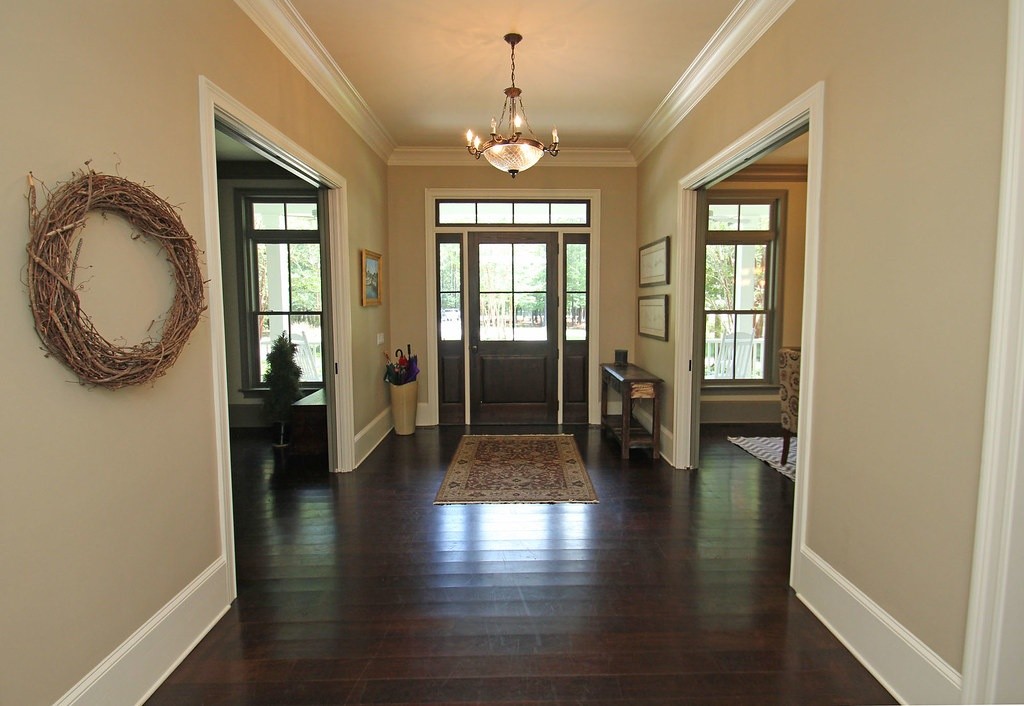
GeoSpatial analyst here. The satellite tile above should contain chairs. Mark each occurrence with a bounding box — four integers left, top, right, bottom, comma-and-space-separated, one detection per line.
777, 347, 803, 466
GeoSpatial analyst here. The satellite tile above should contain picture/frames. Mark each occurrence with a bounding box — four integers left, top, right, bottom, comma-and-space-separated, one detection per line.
639, 235, 670, 288
361, 247, 383, 307
638, 294, 668, 342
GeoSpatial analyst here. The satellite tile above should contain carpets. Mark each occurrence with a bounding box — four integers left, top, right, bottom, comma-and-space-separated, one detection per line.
432, 433, 601, 504
727, 436, 797, 483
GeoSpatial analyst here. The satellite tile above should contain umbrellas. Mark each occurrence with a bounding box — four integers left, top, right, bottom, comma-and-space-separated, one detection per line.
384, 344, 409, 386
405, 344, 419, 382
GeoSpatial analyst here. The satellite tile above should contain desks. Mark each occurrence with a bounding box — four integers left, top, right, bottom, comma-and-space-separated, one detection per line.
600, 362, 665, 459
290, 386, 328, 459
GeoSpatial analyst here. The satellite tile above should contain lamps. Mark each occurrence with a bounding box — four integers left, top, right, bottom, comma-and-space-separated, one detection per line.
465, 33, 561, 179
